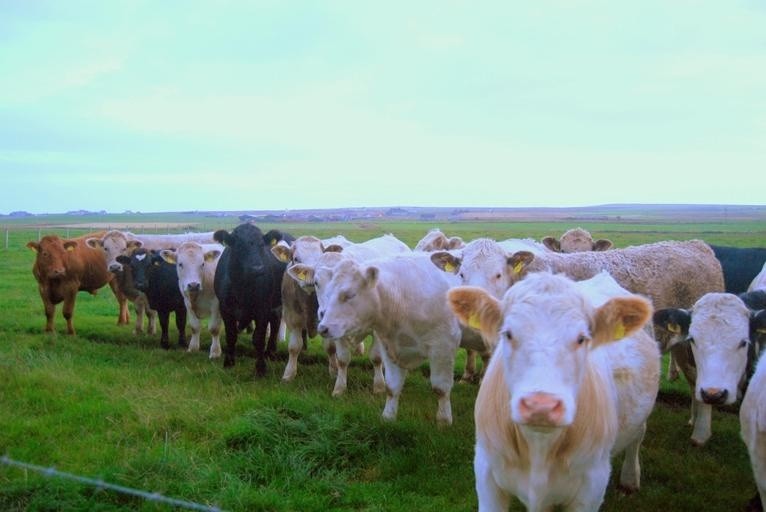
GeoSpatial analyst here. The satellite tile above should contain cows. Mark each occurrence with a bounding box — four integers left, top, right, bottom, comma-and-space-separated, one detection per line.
114, 224, 766, 445
24, 231, 131, 335
84, 230, 232, 327
446, 269, 663, 512
